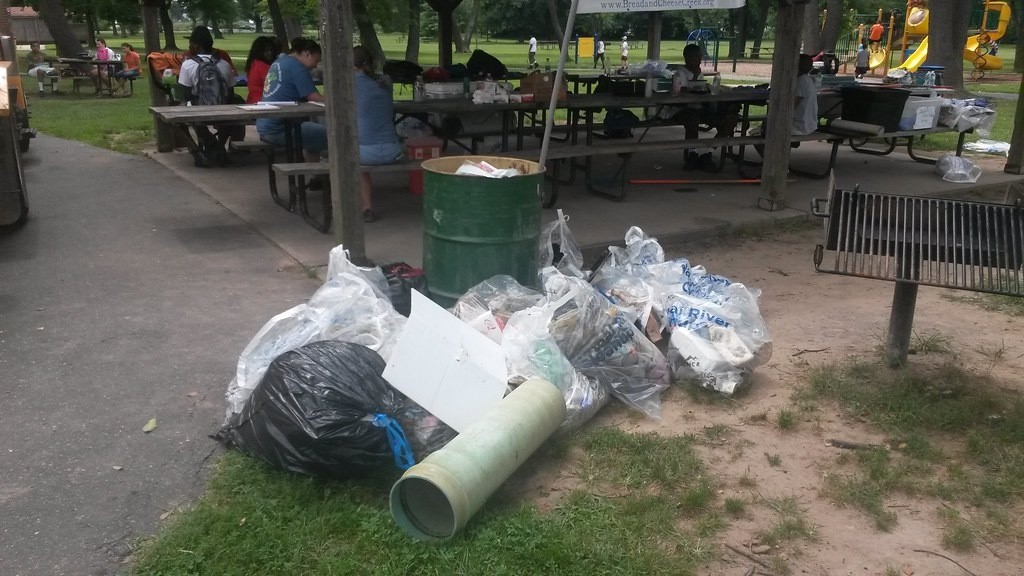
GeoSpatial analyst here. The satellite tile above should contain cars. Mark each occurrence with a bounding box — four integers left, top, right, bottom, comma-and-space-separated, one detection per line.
239, 24, 255, 31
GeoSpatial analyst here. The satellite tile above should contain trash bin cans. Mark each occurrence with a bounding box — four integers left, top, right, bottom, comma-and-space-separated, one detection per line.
916, 66, 945, 86
421, 155, 547, 310
78, 53, 92, 76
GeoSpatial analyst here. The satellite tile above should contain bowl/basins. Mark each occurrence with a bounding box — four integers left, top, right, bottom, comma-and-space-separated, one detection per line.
688, 79, 707, 92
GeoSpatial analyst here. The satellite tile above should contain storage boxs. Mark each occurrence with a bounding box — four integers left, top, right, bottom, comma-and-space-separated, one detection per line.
916, 65, 945, 85
899, 95, 944, 131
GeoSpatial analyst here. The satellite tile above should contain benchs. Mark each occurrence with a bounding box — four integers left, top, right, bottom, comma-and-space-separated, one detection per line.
740, 51, 774, 57
20, 72, 136, 98
230, 115, 975, 233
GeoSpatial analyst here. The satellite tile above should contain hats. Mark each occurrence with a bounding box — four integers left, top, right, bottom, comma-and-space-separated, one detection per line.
182, 31, 213, 49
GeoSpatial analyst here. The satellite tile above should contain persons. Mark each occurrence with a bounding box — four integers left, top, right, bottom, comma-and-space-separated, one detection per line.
90, 38, 114, 93
859, 38, 866, 49
984, 36, 998, 56
854, 44, 870, 79
25, 41, 64, 96
241, 34, 328, 166
526, 31, 539, 69
620, 36, 630, 70
175, 24, 250, 153
868, 20, 885, 53
674, 42, 740, 166
352, 43, 406, 224
111, 42, 142, 99
594, 37, 606, 70
753, 54, 819, 176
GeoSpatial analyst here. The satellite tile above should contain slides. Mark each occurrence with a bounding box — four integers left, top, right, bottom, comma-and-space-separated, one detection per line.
889, 35, 928, 76
865, 45, 886, 68
964, 31, 1003, 70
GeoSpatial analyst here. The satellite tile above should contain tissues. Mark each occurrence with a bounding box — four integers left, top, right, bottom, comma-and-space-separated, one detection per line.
162, 68, 178, 84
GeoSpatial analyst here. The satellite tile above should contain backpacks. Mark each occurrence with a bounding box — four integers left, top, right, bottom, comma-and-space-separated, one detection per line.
188, 54, 229, 105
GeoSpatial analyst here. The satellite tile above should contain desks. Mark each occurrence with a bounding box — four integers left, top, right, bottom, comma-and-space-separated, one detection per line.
149, 88, 957, 210
747, 47, 774, 58
45, 58, 125, 98
387, 71, 720, 133
523, 40, 610, 51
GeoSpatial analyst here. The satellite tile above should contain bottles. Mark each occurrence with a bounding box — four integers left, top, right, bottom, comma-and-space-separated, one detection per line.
604, 57, 611, 76
545, 58, 551, 72
463, 72, 493, 94
414, 76, 425, 102
673, 71, 681, 91
925, 71, 936, 85
815, 72, 823, 94
711, 73, 721, 95
645, 73, 652, 98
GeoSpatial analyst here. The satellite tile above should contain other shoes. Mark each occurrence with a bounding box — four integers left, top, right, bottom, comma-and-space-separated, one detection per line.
683, 151, 700, 170
701, 152, 716, 170
364, 201, 377, 222
40, 91, 44, 97
